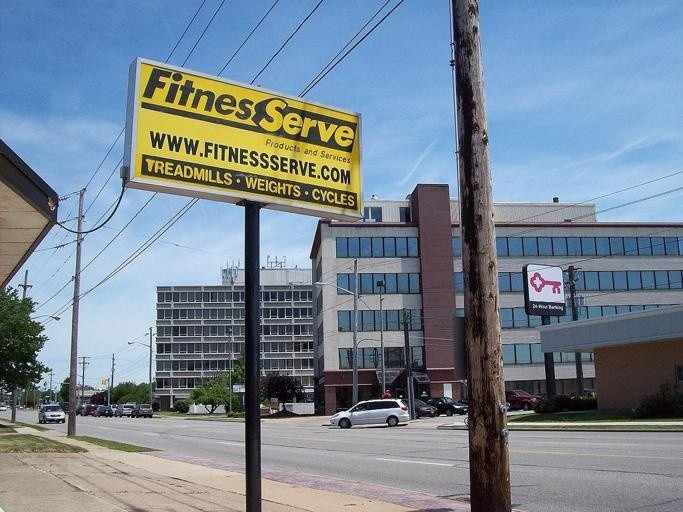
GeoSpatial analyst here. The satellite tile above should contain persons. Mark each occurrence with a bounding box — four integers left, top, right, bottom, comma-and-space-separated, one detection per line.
383, 389, 393, 399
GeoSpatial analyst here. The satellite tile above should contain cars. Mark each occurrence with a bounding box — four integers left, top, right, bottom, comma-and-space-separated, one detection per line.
0, 406, 6, 412
401, 398, 437, 419
505, 389, 536, 410
329, 398, 409, 429
423, 397, 468, 416
75, 404, 153, 418
38, 404, 65, 424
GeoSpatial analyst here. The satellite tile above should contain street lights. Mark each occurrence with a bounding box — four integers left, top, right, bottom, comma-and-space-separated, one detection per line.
128, 329, 152, 404
314, 258, 358, 406
11, 269, 60, 422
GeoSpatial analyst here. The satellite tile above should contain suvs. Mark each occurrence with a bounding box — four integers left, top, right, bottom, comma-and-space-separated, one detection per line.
570, 389, 592, 397
